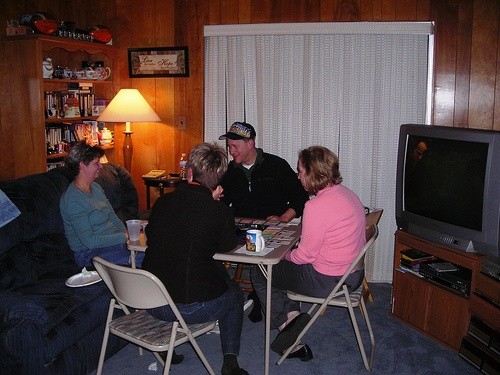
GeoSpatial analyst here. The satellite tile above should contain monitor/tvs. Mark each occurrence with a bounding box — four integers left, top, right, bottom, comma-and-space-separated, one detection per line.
395, 124, 500, 258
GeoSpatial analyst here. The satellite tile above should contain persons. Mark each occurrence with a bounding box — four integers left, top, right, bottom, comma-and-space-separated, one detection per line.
59, 139, 145, 268
251, 146, 364, 352
214, 122, 309, 323
140, 142, 249, 375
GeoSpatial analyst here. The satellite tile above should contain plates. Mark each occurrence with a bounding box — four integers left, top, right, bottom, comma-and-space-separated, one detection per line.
64, 271, 102, 288
179, 153, 187, 180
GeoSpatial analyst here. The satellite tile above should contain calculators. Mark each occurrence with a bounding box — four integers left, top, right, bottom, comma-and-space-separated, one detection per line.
141, 169, 166, 178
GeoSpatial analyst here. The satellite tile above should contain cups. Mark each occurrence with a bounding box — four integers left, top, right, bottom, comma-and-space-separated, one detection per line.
100, 127, 115, 147
140, 221, 148, 241
245, 230, 265, 252
125, 220, 141, 241
169, 172, 180, 177
67, 71, 95, 80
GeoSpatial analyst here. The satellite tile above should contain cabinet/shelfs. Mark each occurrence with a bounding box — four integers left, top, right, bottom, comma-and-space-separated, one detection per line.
458, 272, 500, 375
0, 34, 116, 182
390, 229, 495, 351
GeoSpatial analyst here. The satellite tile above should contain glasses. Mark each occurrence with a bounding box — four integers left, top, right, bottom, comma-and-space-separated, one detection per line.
416, 149, 426, 160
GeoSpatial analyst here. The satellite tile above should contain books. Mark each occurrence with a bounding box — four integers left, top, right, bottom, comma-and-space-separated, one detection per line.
44, 82, 95, 117
45, 121, 105, 145
400, 249, 434, 269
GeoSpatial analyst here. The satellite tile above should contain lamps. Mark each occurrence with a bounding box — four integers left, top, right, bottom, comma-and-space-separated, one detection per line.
96, 88, 162, 174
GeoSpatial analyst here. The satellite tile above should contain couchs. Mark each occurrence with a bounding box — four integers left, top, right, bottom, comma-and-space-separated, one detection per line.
0, 164, 140, 375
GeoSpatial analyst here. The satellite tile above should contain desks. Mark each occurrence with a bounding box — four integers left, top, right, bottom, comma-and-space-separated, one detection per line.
128, 217, 301, 375
142, 176, 178, 209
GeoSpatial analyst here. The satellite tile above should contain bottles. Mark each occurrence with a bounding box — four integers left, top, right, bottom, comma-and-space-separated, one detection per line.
139, 226, 146, 247
6, 12, 95, 43
42, 57, 70, 79
64, 91, 81, 118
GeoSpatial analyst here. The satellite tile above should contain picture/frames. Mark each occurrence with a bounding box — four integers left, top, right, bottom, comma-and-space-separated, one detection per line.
128, 46, 189, 78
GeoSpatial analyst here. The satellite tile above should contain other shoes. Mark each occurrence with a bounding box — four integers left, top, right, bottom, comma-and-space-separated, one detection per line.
157, 349, 184, 365
278, 344, 313, 362
221, 365, 250, 375
271, 313, 311, 353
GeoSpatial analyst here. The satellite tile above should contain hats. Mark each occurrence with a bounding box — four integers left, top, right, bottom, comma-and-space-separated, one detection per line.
218, 121, 257, 141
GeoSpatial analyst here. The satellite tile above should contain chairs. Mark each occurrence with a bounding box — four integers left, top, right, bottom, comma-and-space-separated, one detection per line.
276, 207, 383, 373
92, 256, 216, 375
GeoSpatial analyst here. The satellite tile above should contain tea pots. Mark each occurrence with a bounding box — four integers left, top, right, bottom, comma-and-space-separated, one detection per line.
88, 64, 111, 80
